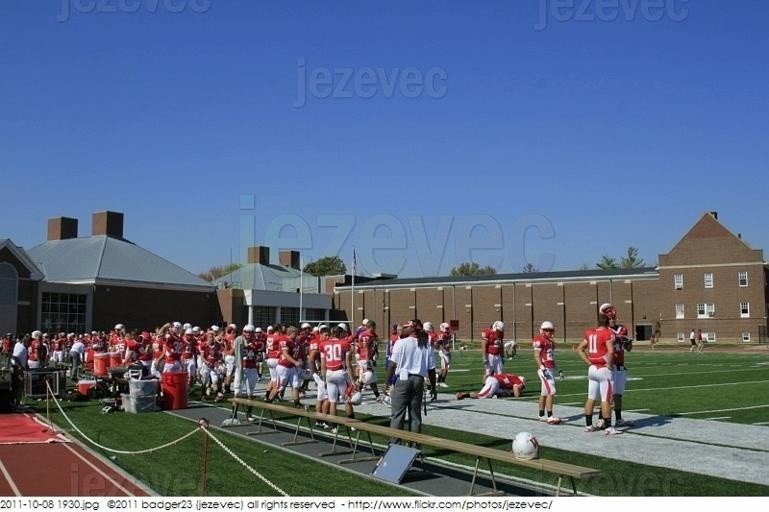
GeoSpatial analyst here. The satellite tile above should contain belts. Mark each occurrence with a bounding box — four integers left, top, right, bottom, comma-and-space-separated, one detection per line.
397, 374, 424, 379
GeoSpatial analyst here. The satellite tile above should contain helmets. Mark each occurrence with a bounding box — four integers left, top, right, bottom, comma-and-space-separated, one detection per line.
420, 322, 434, 336
361, 370, 377, 385
211, 325, 220, 332
599, 302, 616, 316
511, 431, 540, 460
227, 323, 237, 330
266, 325, 275, 335
518, 375, 527, 388
539, 320, 555, 335
440, 322, 450, 333
42, 330, 98, 339
299, 322, 329, 332
338, 322, 347, 331
361, 319, 369, 327
31, 330, 42, 340
114, 323, 125, 335
173, 321, 204, 336
344, 384, 363, 406
254, 327, 263, 335
491, 320, 506, 333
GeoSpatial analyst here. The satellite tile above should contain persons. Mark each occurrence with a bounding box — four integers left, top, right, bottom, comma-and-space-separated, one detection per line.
503, 338, 521, 360
455, 373, 527, 401
531, 319, 564, 425
695, 328, 705, 353
688, 327, 698, 352
576, 313, 624, 437
650, 330, 661, 350
595, 315, 633, 431
480, 319, 506, 385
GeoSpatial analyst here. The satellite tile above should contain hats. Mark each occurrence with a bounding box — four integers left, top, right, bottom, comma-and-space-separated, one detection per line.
402, 320, 416, 328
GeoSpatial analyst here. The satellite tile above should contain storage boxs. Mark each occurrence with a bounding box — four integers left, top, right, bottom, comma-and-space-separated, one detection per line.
119, 377, 158, 414
78, 380, 102, 396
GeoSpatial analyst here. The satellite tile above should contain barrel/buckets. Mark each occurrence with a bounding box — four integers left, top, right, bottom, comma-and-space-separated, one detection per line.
107, 352, 122, 368
94, 353, 111, 376
78, 380, 96, 397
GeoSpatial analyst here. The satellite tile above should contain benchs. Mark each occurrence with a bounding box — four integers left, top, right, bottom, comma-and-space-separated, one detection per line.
229, 398, 363, 456
337, 422, 599, 496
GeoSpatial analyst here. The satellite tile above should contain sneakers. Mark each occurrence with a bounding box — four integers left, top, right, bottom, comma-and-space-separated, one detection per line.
314, 421, 357, 435
201, 389, 256, 423
261, 386, 312, 409
436, 381, 501, 400
538, 414, 635, 435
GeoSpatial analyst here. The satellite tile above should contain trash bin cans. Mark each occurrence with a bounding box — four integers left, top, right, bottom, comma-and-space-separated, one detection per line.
93, 352, 122, 376
128, 380, 157, 413
